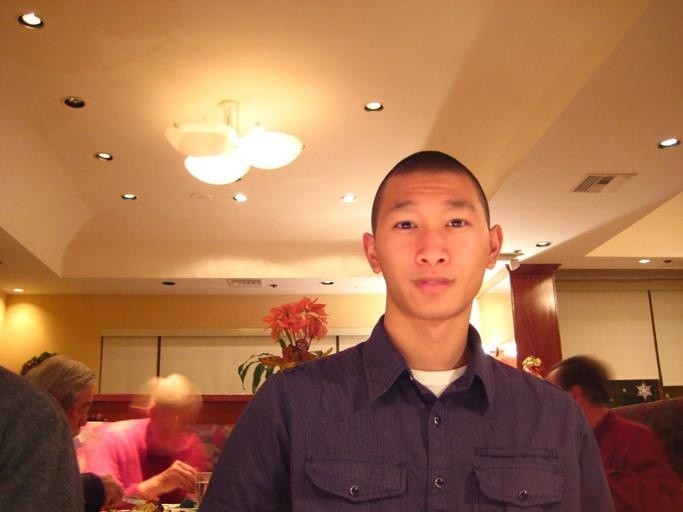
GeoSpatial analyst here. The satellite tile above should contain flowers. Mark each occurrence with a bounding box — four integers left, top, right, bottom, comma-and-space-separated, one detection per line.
233, 296, 333, 394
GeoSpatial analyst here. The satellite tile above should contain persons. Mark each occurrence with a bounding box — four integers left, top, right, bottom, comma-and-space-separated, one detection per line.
82, 372, 213, 503
190, 149, 620, 510
18, 350, 125, 511
544, 352, 683, 510
0, 362, 86, 511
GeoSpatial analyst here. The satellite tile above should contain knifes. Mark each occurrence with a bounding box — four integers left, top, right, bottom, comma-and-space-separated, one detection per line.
120, 498, 146, 505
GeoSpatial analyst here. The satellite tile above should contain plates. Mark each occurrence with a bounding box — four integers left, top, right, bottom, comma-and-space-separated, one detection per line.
161, 503, 198, 512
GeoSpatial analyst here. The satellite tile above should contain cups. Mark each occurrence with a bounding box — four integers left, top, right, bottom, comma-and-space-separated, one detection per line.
195, 471, 212, 512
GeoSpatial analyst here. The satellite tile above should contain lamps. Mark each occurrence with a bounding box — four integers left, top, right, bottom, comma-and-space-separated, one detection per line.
166, 96, 304, 186
